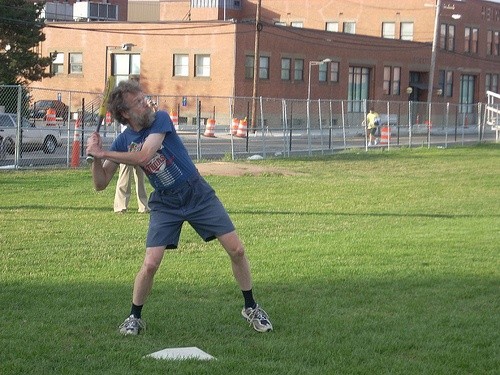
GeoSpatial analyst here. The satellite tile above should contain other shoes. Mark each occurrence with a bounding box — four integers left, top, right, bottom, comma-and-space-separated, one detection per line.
375, 141, 378, 145
368, 143, 372, 146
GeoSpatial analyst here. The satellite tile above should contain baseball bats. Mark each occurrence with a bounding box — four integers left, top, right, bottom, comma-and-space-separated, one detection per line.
86, 76, 117, 163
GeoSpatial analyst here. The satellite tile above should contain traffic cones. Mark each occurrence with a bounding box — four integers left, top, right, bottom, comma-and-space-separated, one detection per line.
464, 112, 469, 129
416, 113, 419, 125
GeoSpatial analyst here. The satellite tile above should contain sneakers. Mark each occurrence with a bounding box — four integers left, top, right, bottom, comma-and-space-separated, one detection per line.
241, 303, 273, 332
119, 314, 144, 335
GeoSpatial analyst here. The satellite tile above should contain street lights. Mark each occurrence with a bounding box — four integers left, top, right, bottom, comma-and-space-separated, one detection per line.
306, 57, 331, 134
406, 87, 413, 101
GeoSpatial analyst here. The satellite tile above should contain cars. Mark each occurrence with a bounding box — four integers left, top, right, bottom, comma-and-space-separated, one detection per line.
0, 112, 64, 159
24, 99, 69, 122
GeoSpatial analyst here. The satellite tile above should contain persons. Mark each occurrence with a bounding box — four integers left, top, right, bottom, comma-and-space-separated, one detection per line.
85, 76, 273, 336
366, 108, 381, 145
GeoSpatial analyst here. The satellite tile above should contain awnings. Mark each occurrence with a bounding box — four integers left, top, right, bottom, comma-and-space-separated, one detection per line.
411, 83, 441, 89
